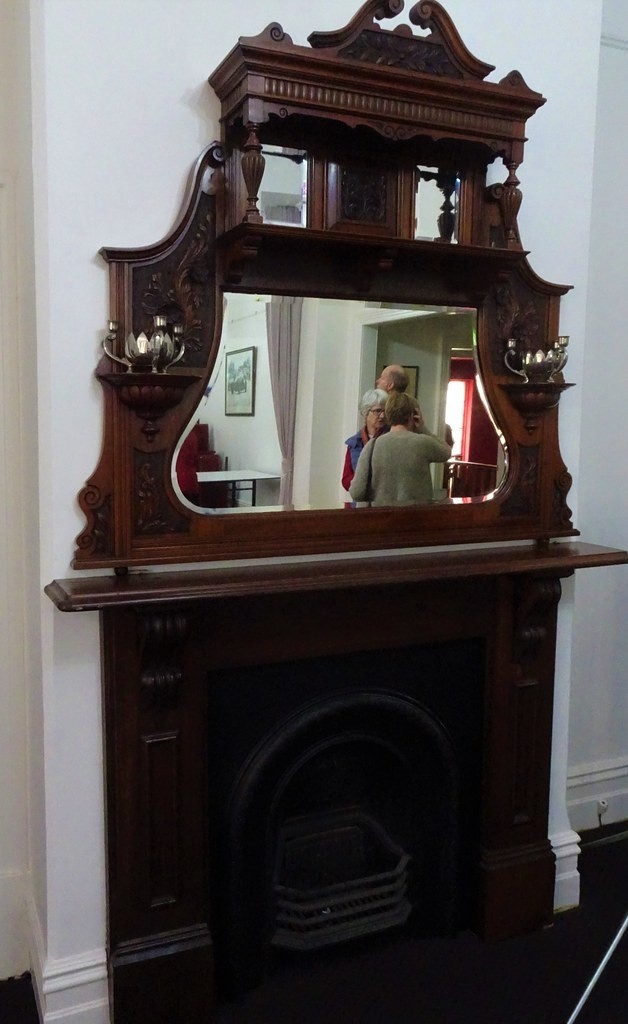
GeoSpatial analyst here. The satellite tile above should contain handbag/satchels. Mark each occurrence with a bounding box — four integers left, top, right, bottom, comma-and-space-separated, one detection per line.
354, 435, 379, 501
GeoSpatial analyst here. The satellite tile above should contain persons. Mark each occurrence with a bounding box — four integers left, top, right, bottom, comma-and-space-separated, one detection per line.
349, 391, 451, 506
342, 389, 389, 509
376, 366, 410, 396
442, 423, 455, 490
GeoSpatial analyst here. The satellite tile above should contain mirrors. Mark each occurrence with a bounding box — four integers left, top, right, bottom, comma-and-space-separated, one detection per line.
170, 284, 510, 513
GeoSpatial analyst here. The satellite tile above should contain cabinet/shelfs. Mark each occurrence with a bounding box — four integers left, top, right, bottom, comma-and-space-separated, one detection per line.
40, 0, 628, 1024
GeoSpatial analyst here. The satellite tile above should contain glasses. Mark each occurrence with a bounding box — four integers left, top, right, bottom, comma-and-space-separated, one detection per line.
369, 409, 385, 416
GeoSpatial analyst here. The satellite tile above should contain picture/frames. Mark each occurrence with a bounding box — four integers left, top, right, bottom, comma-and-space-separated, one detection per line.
224, 346, 255, 417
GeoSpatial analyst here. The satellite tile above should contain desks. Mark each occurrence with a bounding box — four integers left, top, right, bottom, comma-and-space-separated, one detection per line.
197, 472, 280, 506
